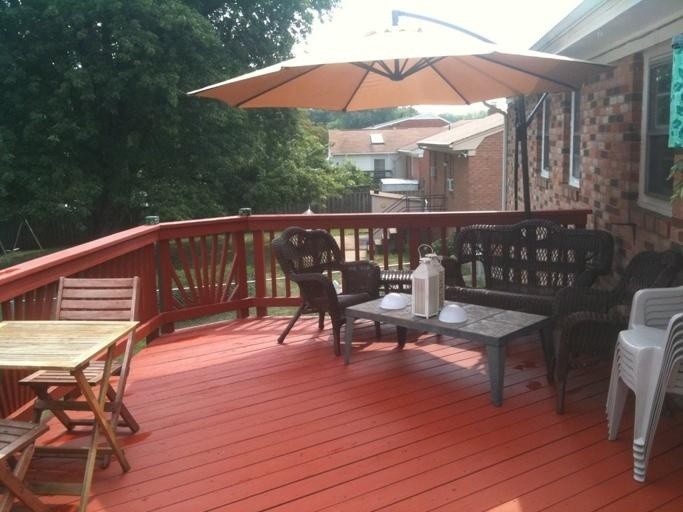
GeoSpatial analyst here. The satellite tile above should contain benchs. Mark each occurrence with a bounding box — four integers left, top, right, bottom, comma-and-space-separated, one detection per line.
386, 219, 618, 378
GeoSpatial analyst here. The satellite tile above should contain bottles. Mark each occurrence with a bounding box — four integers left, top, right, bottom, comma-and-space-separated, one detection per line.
411, 257, 439, 320
424, 253, 446, 313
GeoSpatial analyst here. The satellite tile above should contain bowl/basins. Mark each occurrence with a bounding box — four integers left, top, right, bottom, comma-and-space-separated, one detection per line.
438, 304, 469, 324
379, 292, 407, 310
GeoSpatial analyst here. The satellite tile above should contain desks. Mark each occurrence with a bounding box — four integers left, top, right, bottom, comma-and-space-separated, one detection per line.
0, 320, 141, 512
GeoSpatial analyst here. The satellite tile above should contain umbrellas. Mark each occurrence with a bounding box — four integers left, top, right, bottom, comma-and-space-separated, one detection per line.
188, 10, 617, 288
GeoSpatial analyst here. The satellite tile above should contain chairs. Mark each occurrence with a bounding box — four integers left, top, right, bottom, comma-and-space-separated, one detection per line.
606, 285, 683, 483
0, 419, 48, 512
19, 275, 139, 445
550, 250, 683, 415
271, 226, 381, 356
